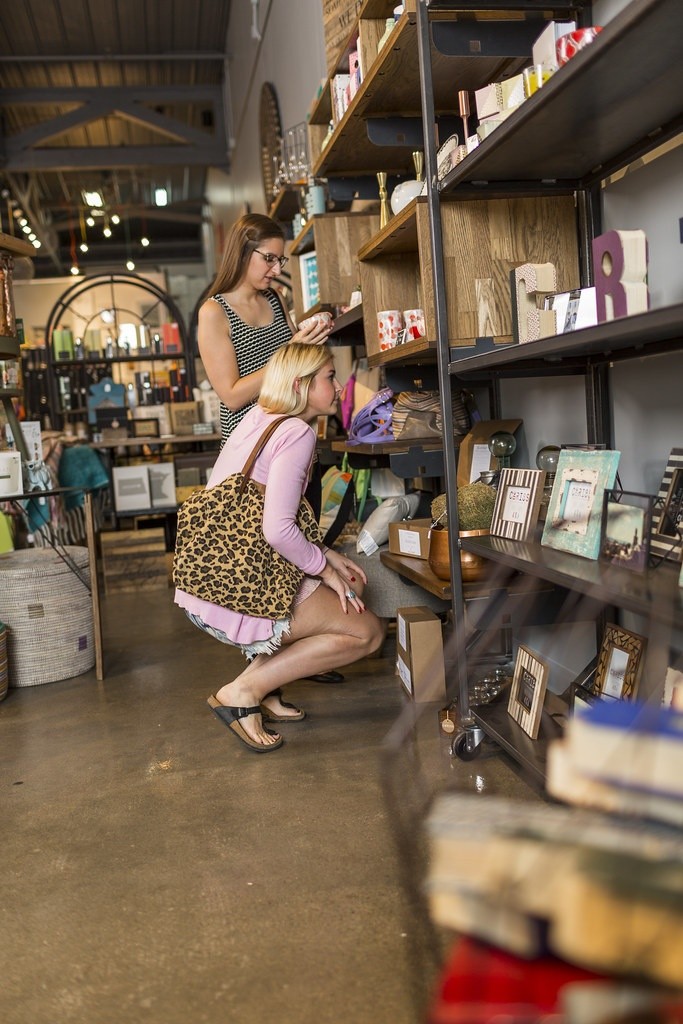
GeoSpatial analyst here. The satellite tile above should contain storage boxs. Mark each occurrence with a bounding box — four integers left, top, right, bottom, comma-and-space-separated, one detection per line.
388, 518, 433, 558
54, 323, 218, 510
395, 606, 446, 702
474, 17, 573, 125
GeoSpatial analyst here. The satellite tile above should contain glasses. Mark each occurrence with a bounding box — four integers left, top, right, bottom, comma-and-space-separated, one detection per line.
253, 248, 289, 269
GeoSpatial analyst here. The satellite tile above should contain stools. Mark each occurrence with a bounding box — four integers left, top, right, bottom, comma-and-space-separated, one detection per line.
340, 542, 448, 659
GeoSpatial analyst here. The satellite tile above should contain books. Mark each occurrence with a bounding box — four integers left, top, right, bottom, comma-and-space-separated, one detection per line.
178, 468, 200, 487
111, 466, 151, 512
531, 19, 576, 66
542, 699, 682, 831
141, 462, 177, 507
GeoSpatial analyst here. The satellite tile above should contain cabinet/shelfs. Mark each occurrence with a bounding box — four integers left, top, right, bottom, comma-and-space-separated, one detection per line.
22, 347, 221, 526
268, 0, 592, 602
417, 0, 683, 789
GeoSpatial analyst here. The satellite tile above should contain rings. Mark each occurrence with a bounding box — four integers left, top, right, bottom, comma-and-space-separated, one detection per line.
348, 592, 356, 599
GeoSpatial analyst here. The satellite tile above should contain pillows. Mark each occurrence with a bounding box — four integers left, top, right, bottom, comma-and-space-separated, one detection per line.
317, 467, 352, 544
356, 489, 421, 553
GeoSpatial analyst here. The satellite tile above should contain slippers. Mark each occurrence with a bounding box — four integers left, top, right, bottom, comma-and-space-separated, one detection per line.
207, 693, 284, 753
259, 688, 306, 724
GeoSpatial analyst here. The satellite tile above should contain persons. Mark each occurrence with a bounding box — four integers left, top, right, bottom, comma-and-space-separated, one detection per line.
171, 341, 387, 754
189, 213, 335, 456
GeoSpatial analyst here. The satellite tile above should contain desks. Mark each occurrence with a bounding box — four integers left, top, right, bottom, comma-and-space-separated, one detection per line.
0, 486, 104, 688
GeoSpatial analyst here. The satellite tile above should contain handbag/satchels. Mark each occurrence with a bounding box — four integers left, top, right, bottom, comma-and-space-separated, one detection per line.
343, 386, 471, 447
171, 415, 326, 620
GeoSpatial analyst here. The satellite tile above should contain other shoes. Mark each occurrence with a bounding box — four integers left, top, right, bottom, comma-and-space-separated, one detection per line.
302, 670, 344, 682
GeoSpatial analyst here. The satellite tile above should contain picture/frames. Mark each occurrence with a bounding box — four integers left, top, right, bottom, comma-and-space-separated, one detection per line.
592, 620, 650, 703
540, 448, 620, 560
569, 682, 602, 718
600, 489, 682, 574
132, 418, 160, 437
507, 646, 549, 741
489, 469, 546, 541
649, 446, 683, 568
